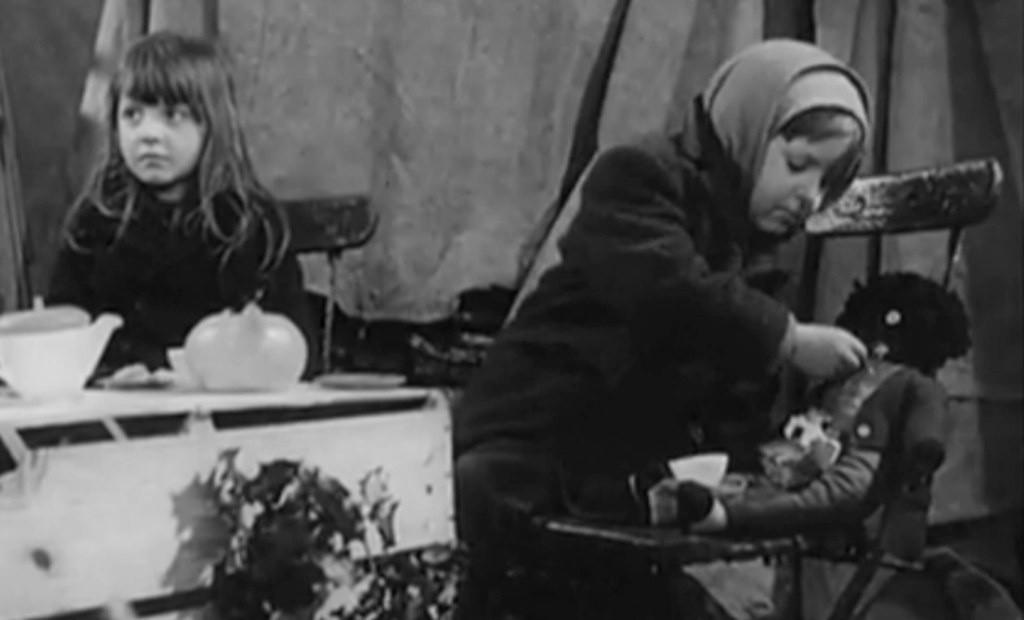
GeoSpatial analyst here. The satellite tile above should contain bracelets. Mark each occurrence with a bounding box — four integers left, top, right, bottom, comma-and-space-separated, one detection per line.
784, 330, 798, 363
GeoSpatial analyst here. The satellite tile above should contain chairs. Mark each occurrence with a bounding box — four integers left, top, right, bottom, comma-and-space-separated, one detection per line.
268, 189, 381, 371
555, 159, 1004, 620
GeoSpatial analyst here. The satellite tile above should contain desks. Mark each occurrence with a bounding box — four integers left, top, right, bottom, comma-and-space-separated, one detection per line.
0, 382, 455, 620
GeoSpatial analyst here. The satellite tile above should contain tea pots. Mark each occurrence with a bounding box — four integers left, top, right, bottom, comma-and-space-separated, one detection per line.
0, 298, 122, 399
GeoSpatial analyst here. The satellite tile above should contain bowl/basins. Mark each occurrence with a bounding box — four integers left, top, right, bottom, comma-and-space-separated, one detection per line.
669, 454, 728, 488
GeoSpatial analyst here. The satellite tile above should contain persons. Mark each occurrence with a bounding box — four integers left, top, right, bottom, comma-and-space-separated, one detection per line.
43, 30, 319, 387
770, 270, 974, 620
454, 40, 873, 619
